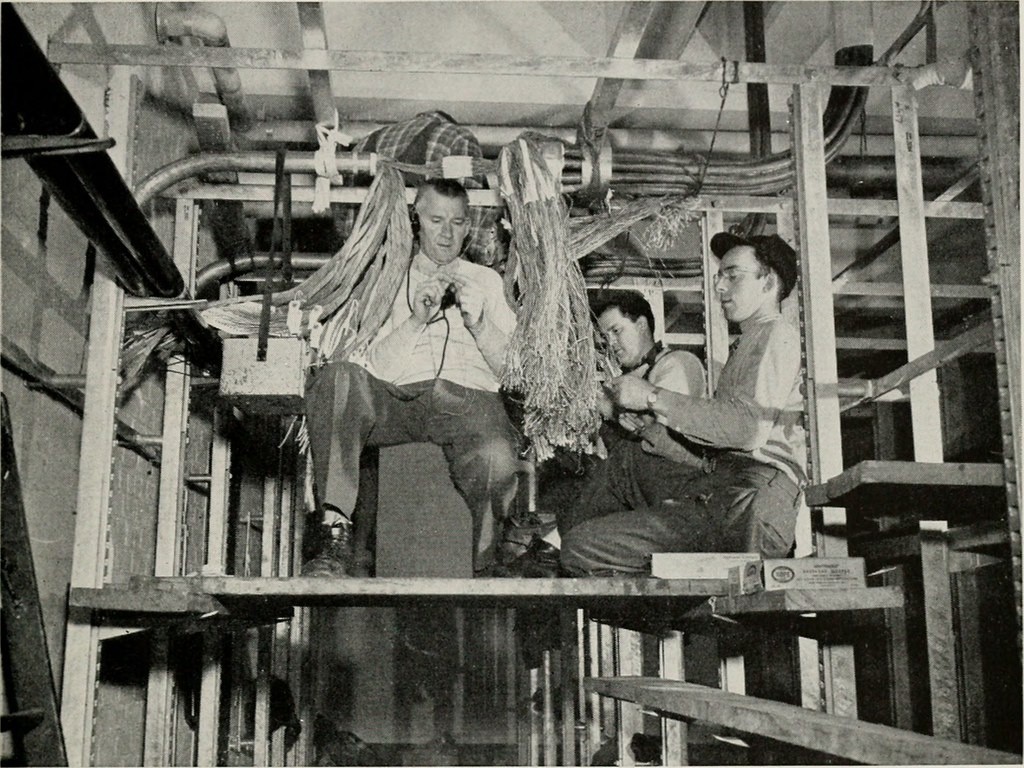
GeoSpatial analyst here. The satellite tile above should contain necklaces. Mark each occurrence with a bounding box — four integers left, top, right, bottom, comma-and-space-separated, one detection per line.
655, 350, 674, 364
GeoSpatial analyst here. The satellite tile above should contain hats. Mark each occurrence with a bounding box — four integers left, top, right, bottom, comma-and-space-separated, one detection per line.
710, 231, 798, 298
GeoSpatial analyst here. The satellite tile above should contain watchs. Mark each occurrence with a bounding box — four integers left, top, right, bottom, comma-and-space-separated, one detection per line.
648, 387, 663, 412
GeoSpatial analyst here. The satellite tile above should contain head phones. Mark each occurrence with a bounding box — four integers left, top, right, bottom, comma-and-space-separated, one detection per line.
410, 207, 421, 237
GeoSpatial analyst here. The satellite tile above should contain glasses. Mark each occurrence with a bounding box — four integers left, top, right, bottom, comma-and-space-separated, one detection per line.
713, 268, 757, 284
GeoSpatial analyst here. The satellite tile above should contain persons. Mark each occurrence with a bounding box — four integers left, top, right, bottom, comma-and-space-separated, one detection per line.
303, 176, 518, 576
507, 233, 806, 578
545, 290, 705, 509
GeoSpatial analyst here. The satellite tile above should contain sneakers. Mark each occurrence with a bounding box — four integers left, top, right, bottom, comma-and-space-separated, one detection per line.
470, 539, 569, 579
300, 519, 357, 580
